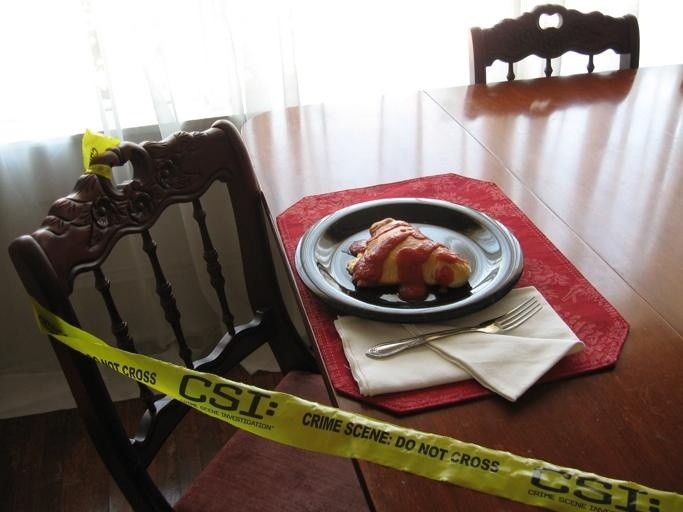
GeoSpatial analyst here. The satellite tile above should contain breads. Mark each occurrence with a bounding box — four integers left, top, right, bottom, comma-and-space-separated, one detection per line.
345, 217, 472, 303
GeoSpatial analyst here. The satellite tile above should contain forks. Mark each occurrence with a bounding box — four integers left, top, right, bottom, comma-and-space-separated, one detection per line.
365, 295, 544, 360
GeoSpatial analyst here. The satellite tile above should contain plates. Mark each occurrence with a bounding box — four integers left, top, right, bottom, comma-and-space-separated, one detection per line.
292, 197, 525, 325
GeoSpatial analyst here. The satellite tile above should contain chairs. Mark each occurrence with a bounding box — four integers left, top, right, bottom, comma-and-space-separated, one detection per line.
7, 117, 373, 511
